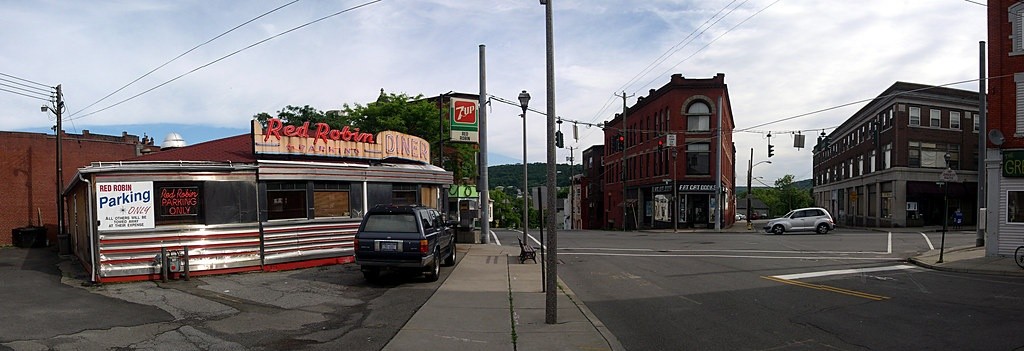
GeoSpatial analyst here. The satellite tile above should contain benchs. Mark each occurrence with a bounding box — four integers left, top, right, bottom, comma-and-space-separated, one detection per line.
516, 235, 537, 265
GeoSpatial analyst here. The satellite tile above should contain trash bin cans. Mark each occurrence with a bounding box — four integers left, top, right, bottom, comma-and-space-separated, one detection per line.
953, 211, 963, 229
12, 226, 48, 249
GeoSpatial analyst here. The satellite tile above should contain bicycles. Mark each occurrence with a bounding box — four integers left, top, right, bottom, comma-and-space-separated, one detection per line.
1014, 246, 1024, 269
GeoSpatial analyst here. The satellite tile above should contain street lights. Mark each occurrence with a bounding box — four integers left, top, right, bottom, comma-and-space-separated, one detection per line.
519, 90, 531, 247
936, 152, 952, 263
41, 104, 64, 235
746, 160, 772, 223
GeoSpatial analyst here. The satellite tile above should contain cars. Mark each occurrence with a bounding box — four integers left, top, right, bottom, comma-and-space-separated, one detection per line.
734, 212, 767, 222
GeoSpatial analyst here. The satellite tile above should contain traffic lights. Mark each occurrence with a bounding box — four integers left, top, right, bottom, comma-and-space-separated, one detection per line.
658, 140, 664, 154
555, 130, 561, 148
618, 134, 625, 150
768, 144, 774, 157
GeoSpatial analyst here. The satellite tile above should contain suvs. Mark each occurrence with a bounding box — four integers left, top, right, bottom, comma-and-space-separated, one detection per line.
354, 200, 459, 282
764, 207, 835, 235
